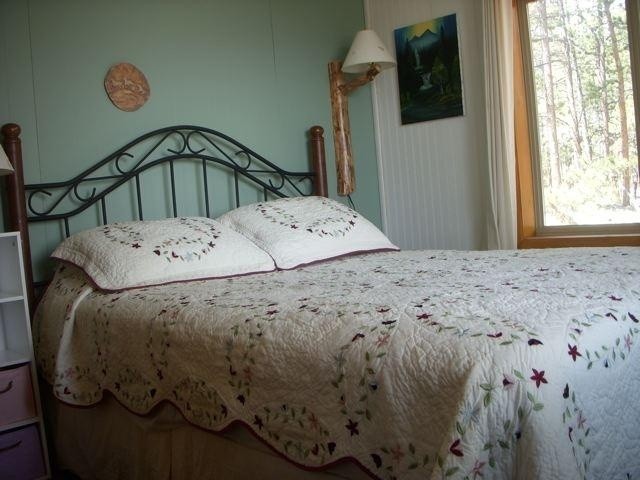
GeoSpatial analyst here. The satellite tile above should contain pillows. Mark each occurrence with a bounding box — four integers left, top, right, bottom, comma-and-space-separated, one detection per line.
217, 196, 401, 269
50, 215, 276, 290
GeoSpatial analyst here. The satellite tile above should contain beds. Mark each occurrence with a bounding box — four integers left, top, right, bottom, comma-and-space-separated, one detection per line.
0, 124, 639, 479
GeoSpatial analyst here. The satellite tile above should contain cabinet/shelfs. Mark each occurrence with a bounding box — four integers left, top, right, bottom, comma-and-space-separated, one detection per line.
0, 230, 53, 480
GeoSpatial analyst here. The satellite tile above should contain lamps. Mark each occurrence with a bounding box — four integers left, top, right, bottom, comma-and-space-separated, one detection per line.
327, 30, 397, 197
0, 143, 15, 176
